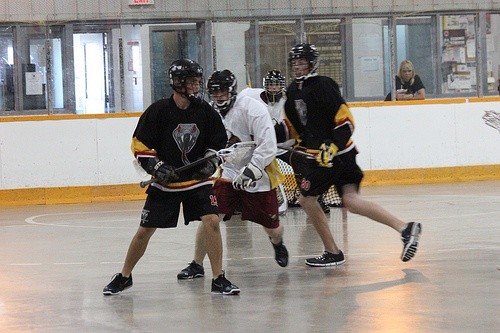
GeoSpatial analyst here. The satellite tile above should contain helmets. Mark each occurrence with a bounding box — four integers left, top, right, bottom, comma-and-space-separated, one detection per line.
168, 59, 203, 105
205, 69, 237, 109
289, 43, 320, 74
263, 70, 285, 94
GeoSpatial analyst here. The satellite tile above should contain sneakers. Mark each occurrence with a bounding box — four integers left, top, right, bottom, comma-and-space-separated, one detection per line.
305, 250, 345, 266
400, 221, 423, 262
211, 273, 240, 295
319, 199, 334, 216
268, 236, 289, 268
177, 259, 205, 279
103, 272, 133, 295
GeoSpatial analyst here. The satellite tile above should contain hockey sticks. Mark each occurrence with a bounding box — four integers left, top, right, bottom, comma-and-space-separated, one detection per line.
276, 144, 316, 158
139, 142, 257, 188
134, 159, 257, 188
273, 161, 289, 215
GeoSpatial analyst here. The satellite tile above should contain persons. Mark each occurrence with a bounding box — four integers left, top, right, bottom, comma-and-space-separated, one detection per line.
235, 68, 297, 216
176, 70, 289, 279
284, 43, 422, 266
384, 59, 426, 101
104, 60, 242, 296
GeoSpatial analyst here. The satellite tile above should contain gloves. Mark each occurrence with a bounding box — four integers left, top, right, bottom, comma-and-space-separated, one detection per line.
156, 164, 180, 183
192, 157, 215, 180
232, 162, 264, 191
317, 140, 338, 168
